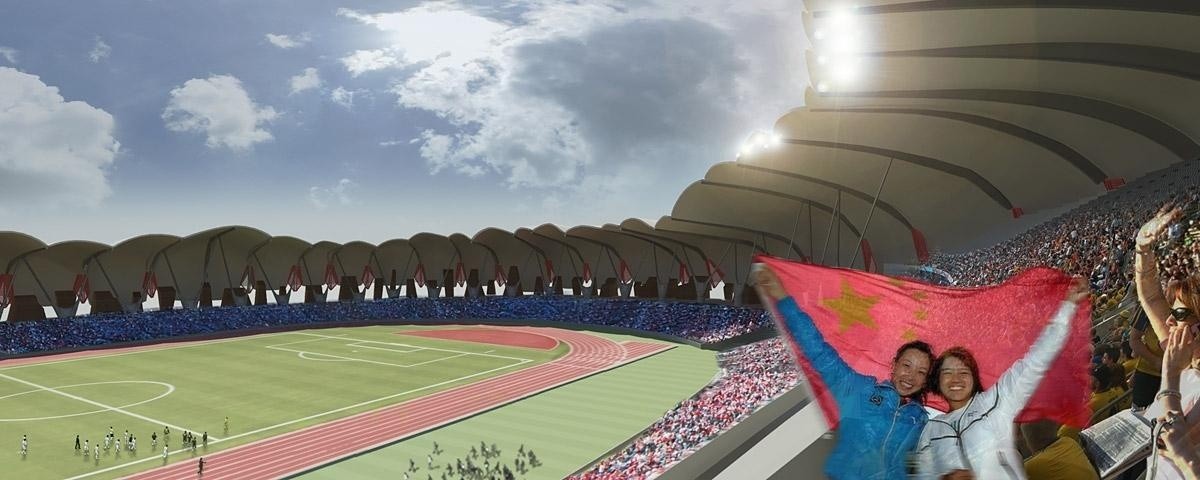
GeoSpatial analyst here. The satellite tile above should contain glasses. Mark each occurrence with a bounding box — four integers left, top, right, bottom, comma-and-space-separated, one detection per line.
1168, 307, 1194, 321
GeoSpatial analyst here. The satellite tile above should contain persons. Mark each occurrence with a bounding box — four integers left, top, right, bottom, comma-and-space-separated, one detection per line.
570, 183, 1198, 478
20, 414, 230, 476
399, 439, 541, 480
0, 295, 571, 360
